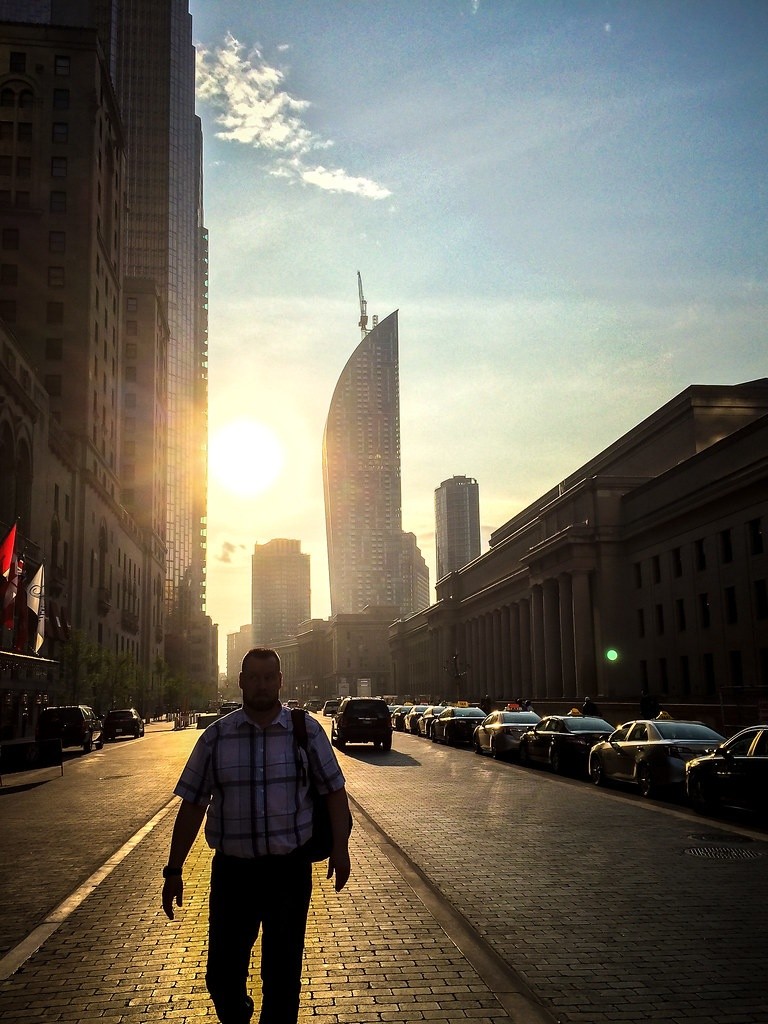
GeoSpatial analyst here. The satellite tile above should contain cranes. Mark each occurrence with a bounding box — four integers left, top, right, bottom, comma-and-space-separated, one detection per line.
355, 270, 379, 337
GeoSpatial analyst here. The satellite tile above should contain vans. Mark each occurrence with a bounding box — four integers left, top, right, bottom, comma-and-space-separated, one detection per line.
35, 704, 106, 753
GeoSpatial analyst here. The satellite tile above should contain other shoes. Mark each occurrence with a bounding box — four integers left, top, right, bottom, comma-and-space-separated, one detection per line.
244, 997, 254, 1024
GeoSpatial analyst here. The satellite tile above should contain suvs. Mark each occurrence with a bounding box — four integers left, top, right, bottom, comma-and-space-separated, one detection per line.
102, 708, 145, 739
331, 696, 393, 752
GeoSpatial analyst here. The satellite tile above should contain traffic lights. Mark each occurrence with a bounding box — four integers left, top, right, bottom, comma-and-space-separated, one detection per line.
605, 646, 621, 664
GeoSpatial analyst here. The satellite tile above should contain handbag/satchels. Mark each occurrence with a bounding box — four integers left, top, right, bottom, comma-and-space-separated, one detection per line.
291, 706, 352, 861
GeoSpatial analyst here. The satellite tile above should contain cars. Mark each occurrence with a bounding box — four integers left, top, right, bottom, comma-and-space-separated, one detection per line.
679, 725, 768, 816
402, 702, 430, 734
518, 706, 617, 775
390, 702, 413, 731
281, 699, 323, 711
417, 705, 454, 737
429, 700, 487, 745
323, 700, 340, 717
474, 703, 544, 759
388, 705, 399, 714
585, 711, 730, 799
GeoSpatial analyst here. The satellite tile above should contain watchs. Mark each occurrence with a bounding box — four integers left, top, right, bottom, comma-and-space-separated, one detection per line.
163, 865, 183, 878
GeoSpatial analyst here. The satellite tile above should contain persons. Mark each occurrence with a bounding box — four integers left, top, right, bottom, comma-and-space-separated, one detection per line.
162, 649, 352, 1024
582, 697, 599, 717
639, 688, 659, 720
514, 698, 534, 711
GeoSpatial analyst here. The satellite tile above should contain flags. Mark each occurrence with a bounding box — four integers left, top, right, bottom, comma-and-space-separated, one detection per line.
26, 563, 45, 653
0, 522, 26, 632
